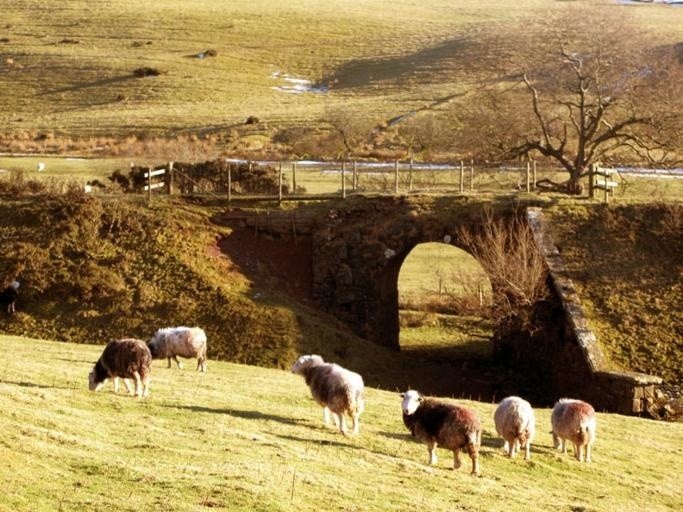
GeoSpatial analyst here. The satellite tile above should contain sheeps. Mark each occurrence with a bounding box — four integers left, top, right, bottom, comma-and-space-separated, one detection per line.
291, 353, 365, 435
494, 394, 535, 461
88, 338, 153, 399
146, 326, 208, 372
398, 388, 482, 477
550, 397, 596, 463
